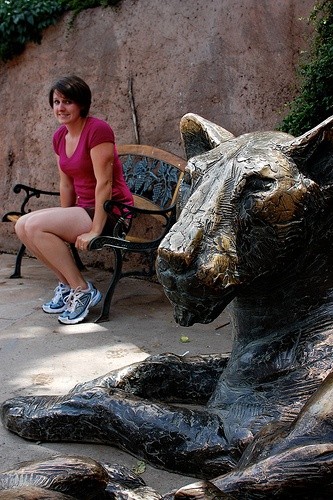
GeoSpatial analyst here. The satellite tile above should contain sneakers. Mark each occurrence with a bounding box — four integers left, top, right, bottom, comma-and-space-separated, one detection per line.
57, 281, 102, 324
42, 282, 73, 313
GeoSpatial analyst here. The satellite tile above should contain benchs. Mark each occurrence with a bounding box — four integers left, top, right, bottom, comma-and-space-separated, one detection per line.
2, 145, 188, 323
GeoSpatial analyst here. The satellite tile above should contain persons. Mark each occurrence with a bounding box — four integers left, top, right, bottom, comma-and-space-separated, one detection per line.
15, 75, 135, 325
